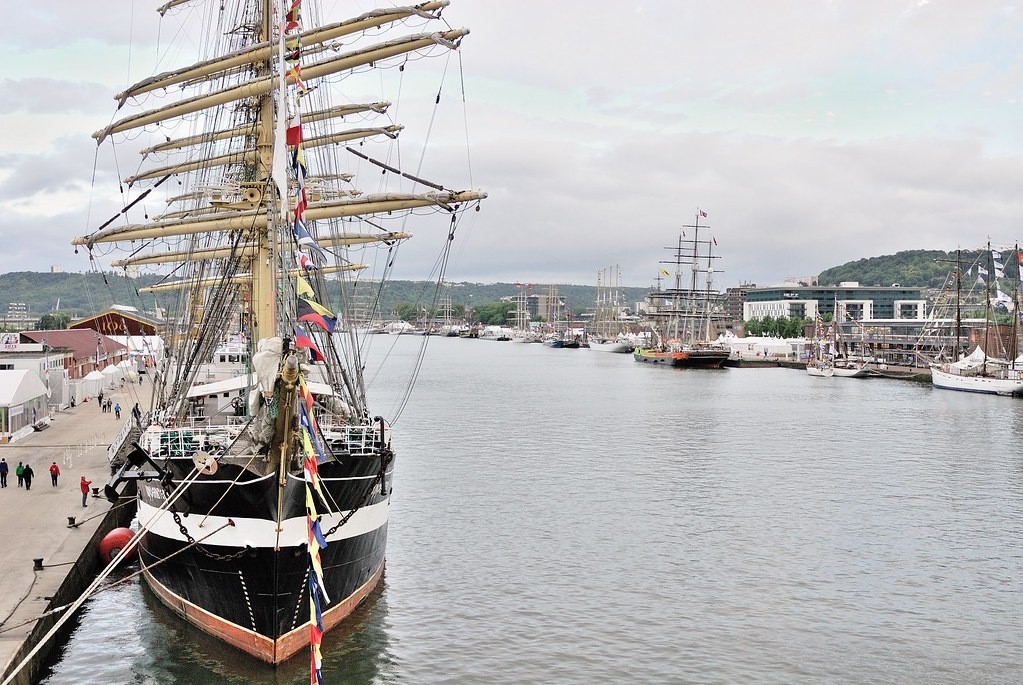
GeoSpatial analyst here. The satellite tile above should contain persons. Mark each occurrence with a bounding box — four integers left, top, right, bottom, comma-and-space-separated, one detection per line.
97, 389, 121, 419
70, 396, 75, 407
139, 376, 142, 385
49, 462, 60, 487
133, 403, 141, 425
81, 477, 92, 507
121, 378, 125, 387
16, 462, 34, 490
0, 458, 8, 488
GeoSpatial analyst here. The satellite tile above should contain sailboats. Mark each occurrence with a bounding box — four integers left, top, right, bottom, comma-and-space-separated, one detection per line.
353, 204, 732, 368
71, 2, 490, 666
806, 234, 1023, 396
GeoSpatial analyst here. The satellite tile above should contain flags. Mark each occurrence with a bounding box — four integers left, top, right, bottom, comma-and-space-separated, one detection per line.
659, 269, 670, 276
809, 302, 862, 369
700, 210, 707, 217
941, 266, 959, 304
295, 295, 338, 685
286, 0, 327, 297
977, 247, 1023, 319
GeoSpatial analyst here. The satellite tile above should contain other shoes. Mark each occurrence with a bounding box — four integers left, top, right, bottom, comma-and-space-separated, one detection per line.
18, 484, 20, 487
5, 485, 7, 487
83, 505, 88, 507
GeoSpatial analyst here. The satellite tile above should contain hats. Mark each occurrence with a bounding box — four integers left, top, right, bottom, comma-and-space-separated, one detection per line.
81, 476, 86, 479
53, 462, 56, 464
